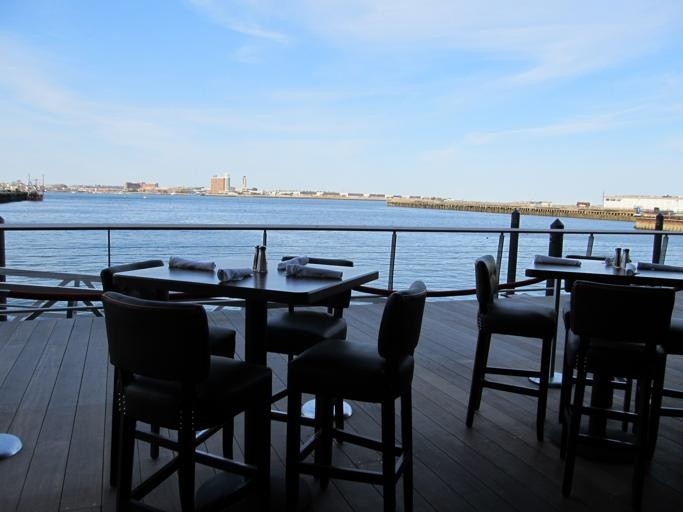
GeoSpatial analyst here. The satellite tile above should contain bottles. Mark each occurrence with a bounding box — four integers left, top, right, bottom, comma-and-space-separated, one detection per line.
251, 245, 260, 272
621, 249, 631, 270
256, 246, 268, 273
613, 247, 622, 269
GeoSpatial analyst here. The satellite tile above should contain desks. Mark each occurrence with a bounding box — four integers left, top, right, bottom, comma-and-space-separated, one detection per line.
525, 251, 683, 457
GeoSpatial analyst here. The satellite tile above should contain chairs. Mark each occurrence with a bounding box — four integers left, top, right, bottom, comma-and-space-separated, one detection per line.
285, 280, 428, 511
100, 250, 379, 487
557, 279, 674, 498
465, 255, 556, 442
102, 291, 272, 510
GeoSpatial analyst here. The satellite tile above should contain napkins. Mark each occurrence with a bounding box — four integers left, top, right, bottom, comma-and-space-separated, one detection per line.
169, 256, 216, 271
637, 261, 682, 273
535, 254, 582, 266
286, 263, 343, 281
217, 267, 254, 281
278, 255, 309, 270
